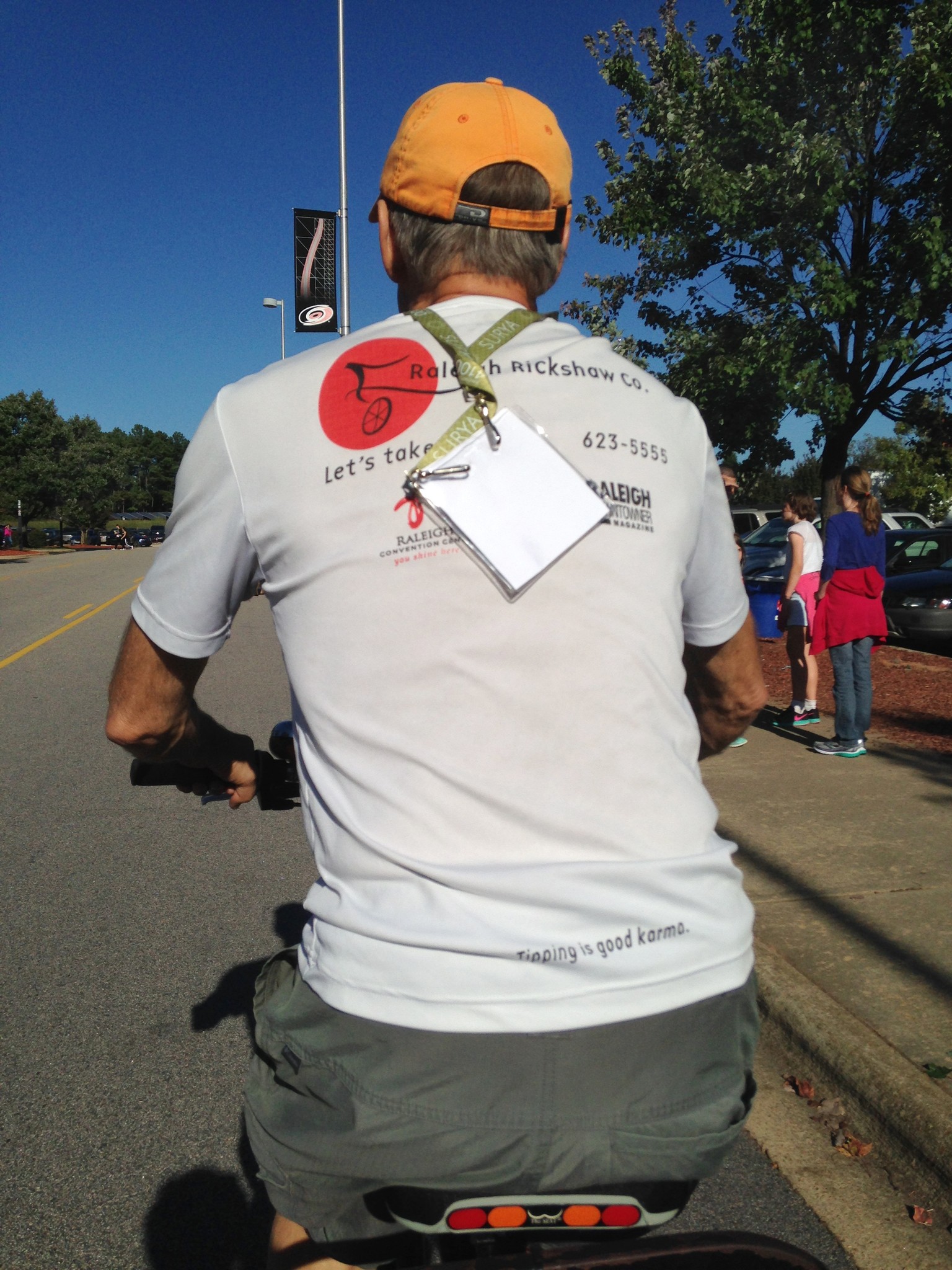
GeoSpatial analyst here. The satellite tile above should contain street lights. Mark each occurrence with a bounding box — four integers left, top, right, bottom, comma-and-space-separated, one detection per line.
263, 298, 286, 361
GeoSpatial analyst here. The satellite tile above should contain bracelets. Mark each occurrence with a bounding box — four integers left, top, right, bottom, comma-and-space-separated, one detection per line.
784, 594, 791, 598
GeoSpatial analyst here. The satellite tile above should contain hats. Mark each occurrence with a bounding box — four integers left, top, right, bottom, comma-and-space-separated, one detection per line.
365, 77, 574, 233
721, 465, 739, 487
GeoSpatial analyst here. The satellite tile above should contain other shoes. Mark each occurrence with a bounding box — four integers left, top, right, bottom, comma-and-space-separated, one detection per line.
131, 545, 133, 550
120, 548, 125, 550
111, 548, 115, 551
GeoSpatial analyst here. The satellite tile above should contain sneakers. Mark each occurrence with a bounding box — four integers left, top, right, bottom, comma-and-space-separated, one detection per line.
773, 706, 810, 726
804, 709, 820, 724
729, 736, 746, 747
813, 736, 867, 758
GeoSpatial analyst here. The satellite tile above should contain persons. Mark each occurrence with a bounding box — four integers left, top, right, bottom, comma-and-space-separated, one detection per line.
103, 79, 767, 1254
778, 490, 822, 729
718, 465, 737, 501
728, 533, 753, 748
812, 468, 885, 759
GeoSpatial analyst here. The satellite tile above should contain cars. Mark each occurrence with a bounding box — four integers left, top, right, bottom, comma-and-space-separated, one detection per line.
0, 524, 165, 547
732, 507, 952, 639
